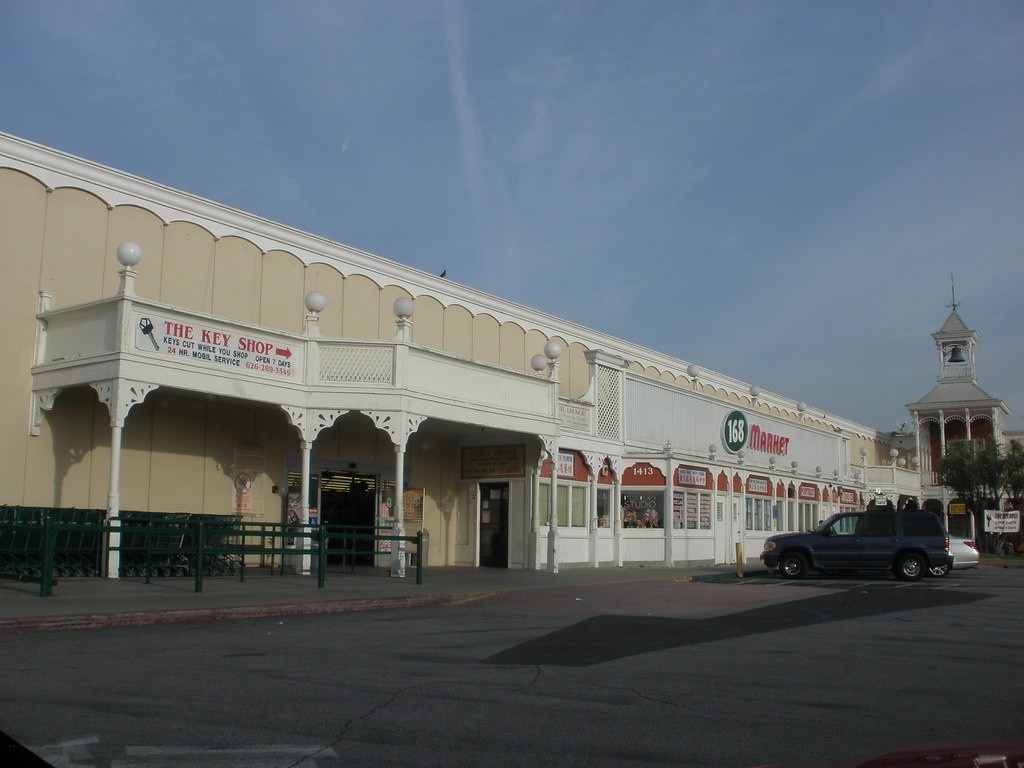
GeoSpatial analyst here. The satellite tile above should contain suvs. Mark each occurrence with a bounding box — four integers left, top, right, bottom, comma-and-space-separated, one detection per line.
760, 508, 951, 582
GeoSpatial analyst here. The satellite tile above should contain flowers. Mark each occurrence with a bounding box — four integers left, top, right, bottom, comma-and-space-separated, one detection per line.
624, 508, 659, 527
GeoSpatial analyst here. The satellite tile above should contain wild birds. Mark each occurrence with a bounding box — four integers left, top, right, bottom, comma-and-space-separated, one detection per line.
440, 270, 447, 278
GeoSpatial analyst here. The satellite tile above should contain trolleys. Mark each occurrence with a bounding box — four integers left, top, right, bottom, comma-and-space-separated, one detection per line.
0, 503, 245, 580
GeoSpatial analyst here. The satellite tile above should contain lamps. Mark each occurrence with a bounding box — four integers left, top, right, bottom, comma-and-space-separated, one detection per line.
768, 457, 776, 472
832, 469, 839, 481
708, 444, 717, 461
790, 461, 799, 474
816, 466, 823, 479
531, 354, 547, 375
861, 447, 869, 465
854, 472, 862, 485
300, 291, 327, 337
113, 241, 143, 297
392, 296, 415, 341
898, 458, 906, 468
737, 450, 745, 465
544, 341, 561, 380
889, 448, 899, 466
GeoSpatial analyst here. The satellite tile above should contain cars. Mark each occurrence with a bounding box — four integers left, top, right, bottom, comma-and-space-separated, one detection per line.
922, 534, 981, 578
